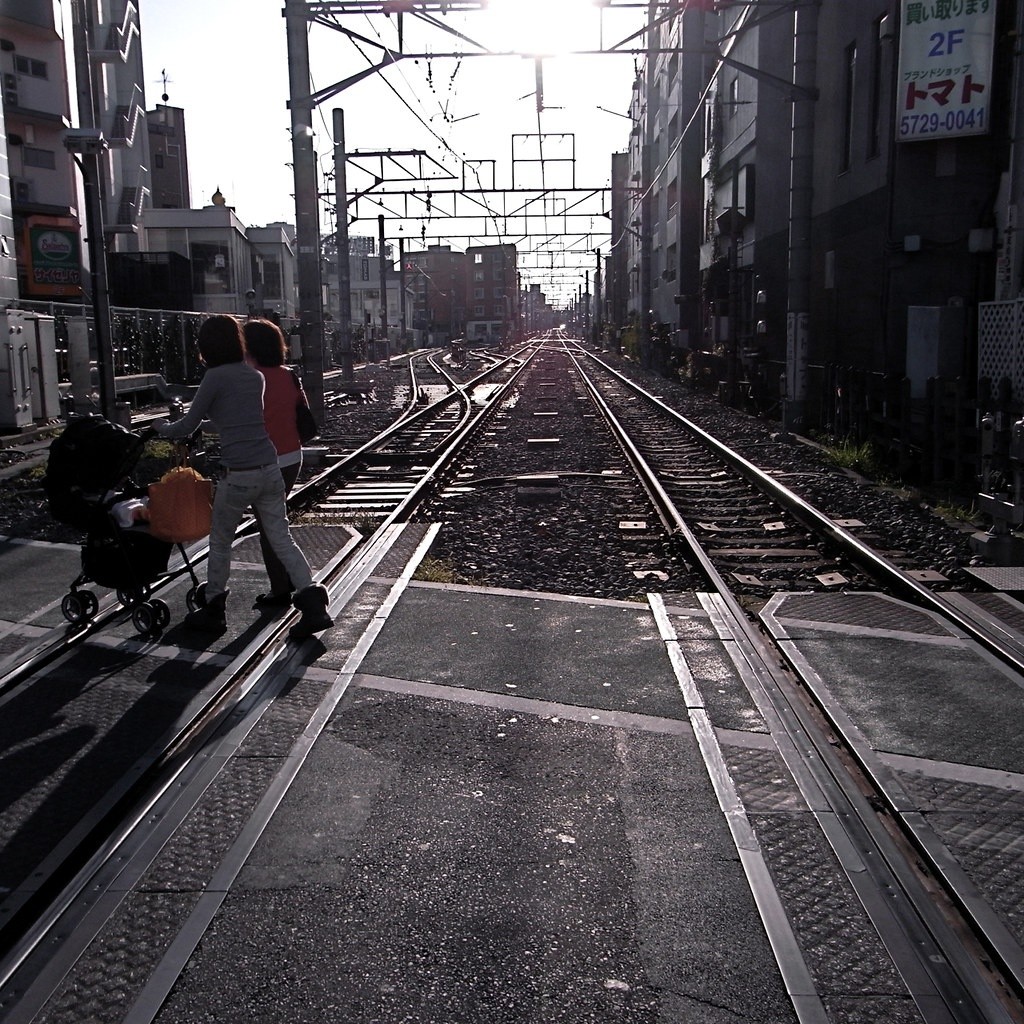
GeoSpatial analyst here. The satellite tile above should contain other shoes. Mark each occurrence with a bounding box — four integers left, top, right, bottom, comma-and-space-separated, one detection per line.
256, 582, 296, 607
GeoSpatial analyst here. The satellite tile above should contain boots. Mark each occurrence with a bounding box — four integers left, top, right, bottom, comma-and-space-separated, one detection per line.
185, 581, 228, 633
289, 585, 334, 637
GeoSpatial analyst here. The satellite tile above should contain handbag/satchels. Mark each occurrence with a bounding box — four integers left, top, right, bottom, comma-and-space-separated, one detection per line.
147, 444, 213, 543
292, 372, 317, 444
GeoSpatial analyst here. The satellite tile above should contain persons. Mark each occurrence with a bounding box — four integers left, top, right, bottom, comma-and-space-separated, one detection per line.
152, 315, 335, 638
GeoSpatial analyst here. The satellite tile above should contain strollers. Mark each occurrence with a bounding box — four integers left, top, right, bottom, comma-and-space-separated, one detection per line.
62, 416, 232, 637
